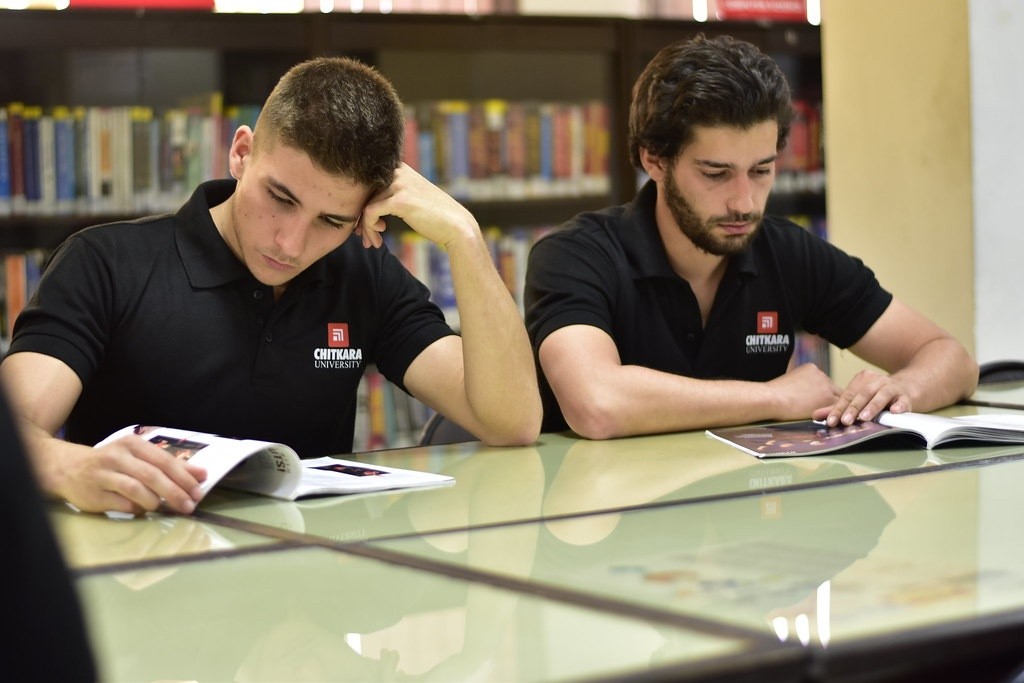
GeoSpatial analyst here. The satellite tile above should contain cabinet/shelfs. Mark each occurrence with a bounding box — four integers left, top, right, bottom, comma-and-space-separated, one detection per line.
1, 9, 828, 452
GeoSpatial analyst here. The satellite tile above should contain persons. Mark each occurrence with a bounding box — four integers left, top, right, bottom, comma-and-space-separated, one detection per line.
519, 31, 982, 450
0, 55, 547, 520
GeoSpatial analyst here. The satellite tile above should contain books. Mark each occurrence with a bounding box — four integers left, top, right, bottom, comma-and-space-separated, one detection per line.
352, 100, 615, 465
0, 98, 265, 427
82, 424, 453, 519
763, 101, 833, 386
704, 403, 1024, 462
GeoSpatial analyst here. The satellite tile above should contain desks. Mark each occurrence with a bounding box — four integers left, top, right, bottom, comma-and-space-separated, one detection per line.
43, 372, 1024, 683
